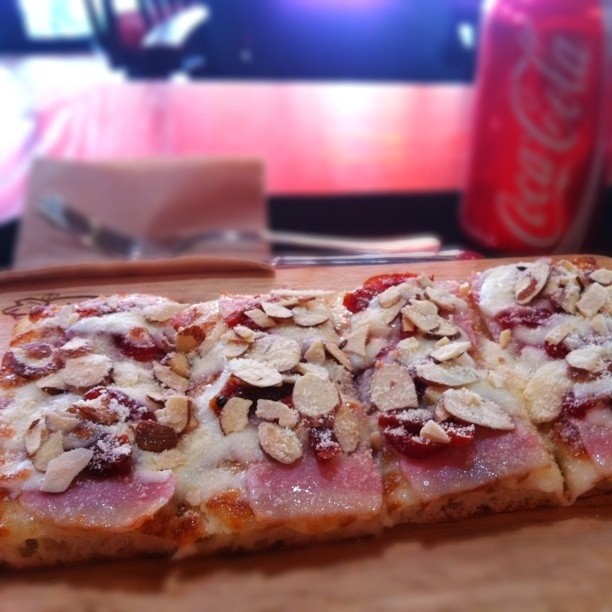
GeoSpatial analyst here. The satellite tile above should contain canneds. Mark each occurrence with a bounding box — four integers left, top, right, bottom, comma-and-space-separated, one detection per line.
455, 0, 612, 253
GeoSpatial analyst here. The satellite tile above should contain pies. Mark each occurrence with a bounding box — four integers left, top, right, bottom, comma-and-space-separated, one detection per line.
0, 255, 611, 574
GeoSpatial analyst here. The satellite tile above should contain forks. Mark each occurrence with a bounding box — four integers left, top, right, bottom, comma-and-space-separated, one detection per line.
35, 191, 441, 254
